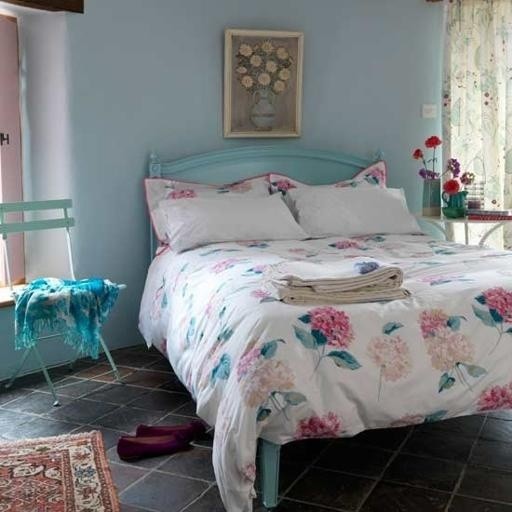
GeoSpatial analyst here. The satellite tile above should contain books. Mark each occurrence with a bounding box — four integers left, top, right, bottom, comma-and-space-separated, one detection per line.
465, 208, 512, 220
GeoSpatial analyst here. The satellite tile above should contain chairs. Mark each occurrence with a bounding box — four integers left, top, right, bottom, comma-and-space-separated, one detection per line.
0, 199, 127, 404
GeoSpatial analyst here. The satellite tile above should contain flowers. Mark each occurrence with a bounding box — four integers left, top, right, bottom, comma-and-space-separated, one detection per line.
411, 136, 442, 181
443, 158, 476, 194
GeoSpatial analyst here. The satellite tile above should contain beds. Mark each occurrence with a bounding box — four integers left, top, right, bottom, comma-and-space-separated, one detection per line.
146, 148, 511, 509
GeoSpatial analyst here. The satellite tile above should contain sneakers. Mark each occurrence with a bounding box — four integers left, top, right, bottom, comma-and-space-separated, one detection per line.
118, 434, 191, 463
136, 421, 205, 441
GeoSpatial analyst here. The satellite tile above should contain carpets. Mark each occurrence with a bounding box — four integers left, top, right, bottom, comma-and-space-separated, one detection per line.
0, 431, 120, 512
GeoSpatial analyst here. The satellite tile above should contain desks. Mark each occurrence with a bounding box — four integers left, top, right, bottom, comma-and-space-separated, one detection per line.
413, 212, 511, 247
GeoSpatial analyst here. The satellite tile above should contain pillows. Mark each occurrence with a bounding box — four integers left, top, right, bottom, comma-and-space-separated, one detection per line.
158, 192, 309, 254
286, 186, 423, 237
269, 161, 387, 220
145, 173, 270, 252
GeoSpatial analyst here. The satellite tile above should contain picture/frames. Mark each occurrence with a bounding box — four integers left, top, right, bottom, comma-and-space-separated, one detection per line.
224, 29, 304, 139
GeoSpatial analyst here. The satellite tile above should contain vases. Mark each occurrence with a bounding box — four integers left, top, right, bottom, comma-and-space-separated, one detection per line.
423, 180, 442, 216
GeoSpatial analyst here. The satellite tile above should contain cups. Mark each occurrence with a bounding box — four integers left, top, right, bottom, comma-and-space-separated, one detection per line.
468, 201, 481, 209
